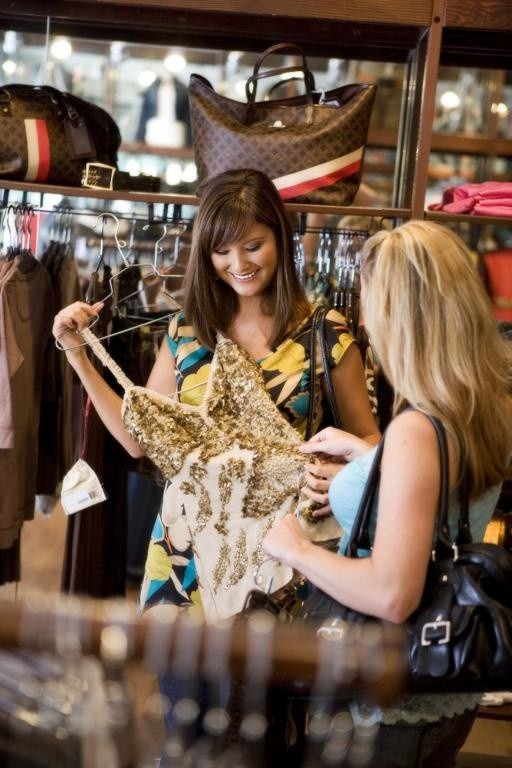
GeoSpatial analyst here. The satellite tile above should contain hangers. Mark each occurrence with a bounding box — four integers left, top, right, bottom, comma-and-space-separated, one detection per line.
0, 196, 374, 326
52, 212, 192, 352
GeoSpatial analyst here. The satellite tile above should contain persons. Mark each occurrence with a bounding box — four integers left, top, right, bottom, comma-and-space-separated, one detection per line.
262, 220, 511, 768
51, 169, 381, 768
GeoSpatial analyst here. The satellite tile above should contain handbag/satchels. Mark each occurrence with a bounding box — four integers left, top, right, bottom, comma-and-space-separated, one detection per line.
188, 40, 376, 208
295, 407, 509, 694
1, 81, 121, 181
302, 303, 344, 443
